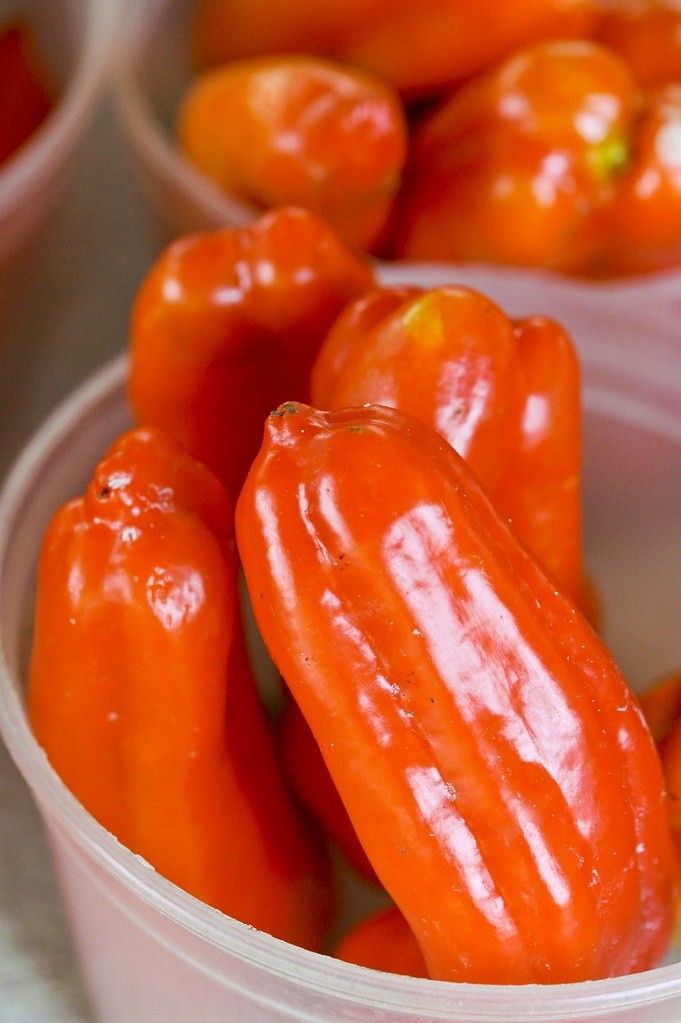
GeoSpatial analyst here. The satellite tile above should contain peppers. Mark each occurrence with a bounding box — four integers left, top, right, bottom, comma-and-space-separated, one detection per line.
0, 0, 681, 989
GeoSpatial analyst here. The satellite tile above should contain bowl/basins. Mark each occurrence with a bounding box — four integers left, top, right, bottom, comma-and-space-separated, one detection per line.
120, 0, 681, 400
0, 1, 115, 271
0, 268, 680, 1022
